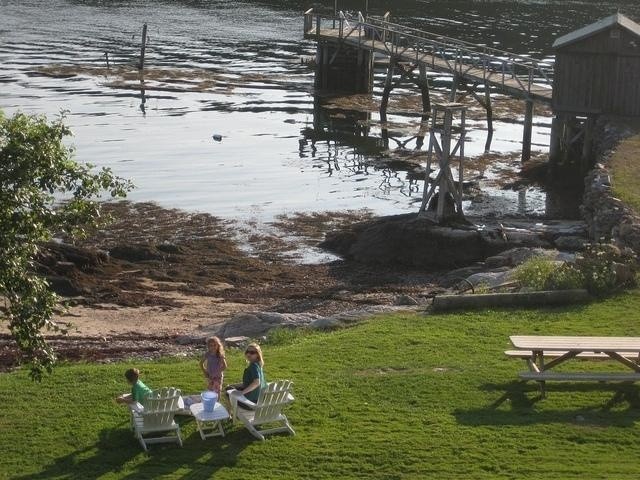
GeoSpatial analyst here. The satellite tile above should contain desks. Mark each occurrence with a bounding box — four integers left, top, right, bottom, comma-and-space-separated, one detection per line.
190, 402, 229, 440
509, 335, 640, 398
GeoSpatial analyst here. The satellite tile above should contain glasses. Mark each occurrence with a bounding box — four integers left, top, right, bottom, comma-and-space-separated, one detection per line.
245, 349, 256, 355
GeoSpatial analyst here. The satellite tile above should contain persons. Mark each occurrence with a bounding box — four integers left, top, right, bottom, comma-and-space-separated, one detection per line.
198, 336, 227, 403
225, 343, 264, 421
116, 368, 161, 410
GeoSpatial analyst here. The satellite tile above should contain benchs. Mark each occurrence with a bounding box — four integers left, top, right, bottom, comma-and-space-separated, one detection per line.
518, 371, 640, 381
503, 350, 639, 358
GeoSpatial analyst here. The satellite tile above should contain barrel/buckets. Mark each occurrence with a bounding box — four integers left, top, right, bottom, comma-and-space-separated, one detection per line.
200, 390, 219, 413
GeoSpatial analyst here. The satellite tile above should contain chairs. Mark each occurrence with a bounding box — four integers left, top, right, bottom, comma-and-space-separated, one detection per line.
123, 387, 182, 451
226, 380, 295, 439
